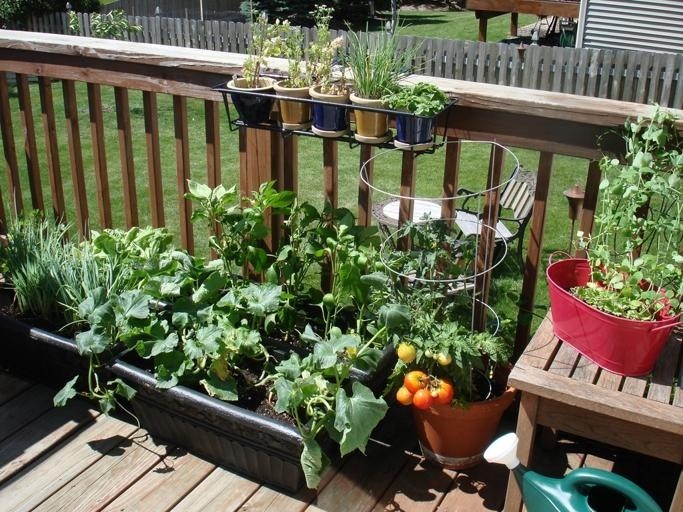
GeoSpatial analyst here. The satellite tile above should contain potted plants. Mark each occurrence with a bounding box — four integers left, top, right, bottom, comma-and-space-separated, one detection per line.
272, 3, 336, 130
310, 36, 356, 140
545, 101, 683, 378
5, 214, 128, 398
183, 179, 398, 401
106, 226, 361, 504
391, 82, 451, 152
226, 11, 288, 126
383, 211, 519, 469
346, 11, 423, 145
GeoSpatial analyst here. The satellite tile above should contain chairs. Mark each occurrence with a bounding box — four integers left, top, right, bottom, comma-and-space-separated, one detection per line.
455, 163, 537, 274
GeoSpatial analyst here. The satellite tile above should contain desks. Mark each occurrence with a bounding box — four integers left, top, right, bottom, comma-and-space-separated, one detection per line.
370, 196, 442, 249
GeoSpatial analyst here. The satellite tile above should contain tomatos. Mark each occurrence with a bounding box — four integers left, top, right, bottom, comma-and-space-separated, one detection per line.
396, 343, 453, 409
